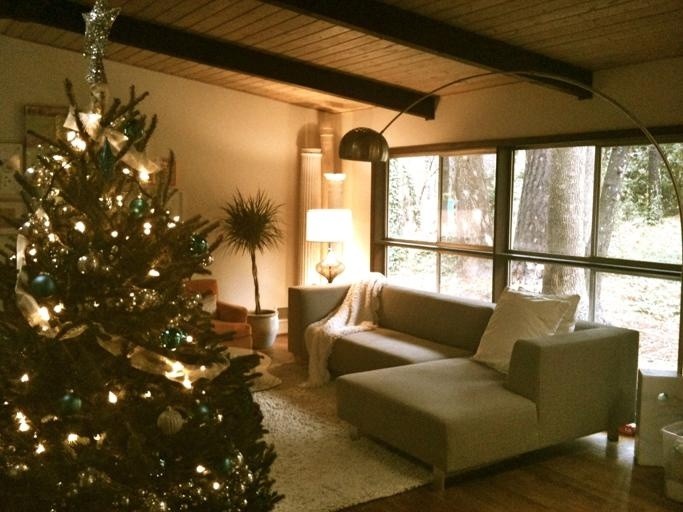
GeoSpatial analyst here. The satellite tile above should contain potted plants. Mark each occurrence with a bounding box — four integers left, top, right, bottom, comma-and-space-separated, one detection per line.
218, 187, 287, 348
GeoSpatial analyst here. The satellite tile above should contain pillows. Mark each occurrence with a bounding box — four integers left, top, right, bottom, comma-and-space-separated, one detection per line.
230, 350, 282, 392
472, 286, 580, 374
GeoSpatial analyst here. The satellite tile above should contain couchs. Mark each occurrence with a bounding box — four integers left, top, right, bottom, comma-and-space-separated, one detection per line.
288, 283, 640, 490
183, 279, 252, 343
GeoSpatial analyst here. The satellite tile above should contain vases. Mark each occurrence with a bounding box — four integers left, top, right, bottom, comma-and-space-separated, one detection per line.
662, 421, 683, 502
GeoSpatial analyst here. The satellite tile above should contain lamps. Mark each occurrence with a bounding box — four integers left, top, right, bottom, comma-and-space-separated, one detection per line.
306, 209, 353, 284
338, 69, 682, 465
324, 173, 346, 183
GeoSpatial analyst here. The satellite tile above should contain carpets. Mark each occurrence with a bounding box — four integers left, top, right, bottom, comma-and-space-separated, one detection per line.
246, 360, 435, 512
